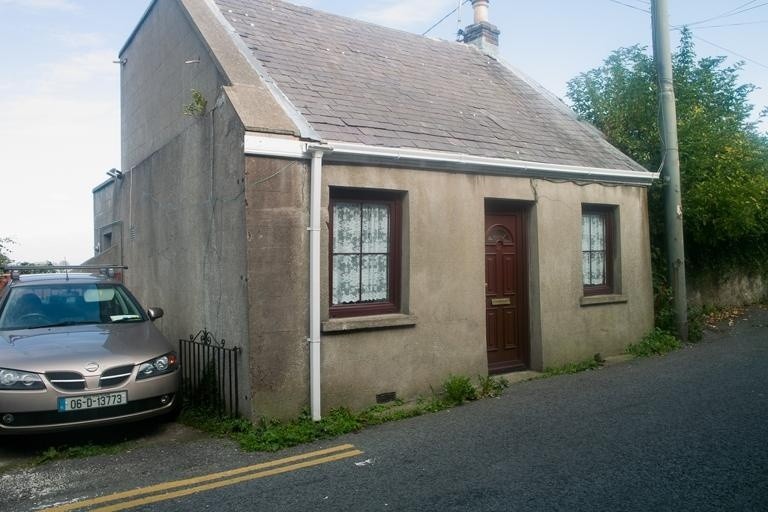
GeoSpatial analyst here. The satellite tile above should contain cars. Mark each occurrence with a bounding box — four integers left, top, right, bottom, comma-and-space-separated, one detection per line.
0, 264, 182, 444
